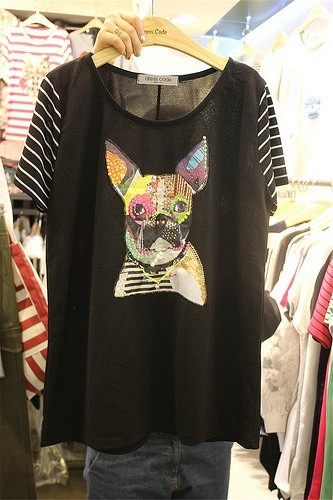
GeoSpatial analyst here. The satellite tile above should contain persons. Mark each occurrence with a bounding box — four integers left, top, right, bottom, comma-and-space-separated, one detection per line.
77, 11, 283, 500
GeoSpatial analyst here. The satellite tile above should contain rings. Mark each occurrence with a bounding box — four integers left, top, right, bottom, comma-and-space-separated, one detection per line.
114, 27, 124, 37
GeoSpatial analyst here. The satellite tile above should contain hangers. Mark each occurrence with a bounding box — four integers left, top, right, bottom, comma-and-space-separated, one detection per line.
14, 7, 106, 39
88, 2, 230, 77
279, 181, 333, 227
13, 211, 46, 240
